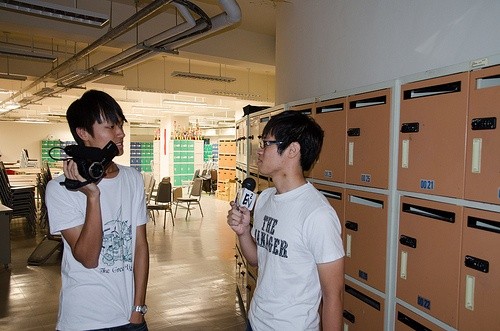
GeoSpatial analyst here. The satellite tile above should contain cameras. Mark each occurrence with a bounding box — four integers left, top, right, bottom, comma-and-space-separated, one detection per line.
63, 140, 120, 183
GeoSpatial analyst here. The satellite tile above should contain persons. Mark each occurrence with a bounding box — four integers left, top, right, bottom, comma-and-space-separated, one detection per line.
227, 110, 346, 331
45, 90, 149, 331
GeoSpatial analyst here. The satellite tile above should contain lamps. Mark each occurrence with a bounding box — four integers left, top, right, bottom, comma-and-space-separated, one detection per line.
123, 55, 259, 129
0, 57, 27, 80
0, 32, 59, 62
0, 0, 110, 27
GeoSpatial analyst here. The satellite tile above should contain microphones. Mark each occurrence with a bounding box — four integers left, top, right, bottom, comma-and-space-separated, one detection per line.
235, 178, 258, 211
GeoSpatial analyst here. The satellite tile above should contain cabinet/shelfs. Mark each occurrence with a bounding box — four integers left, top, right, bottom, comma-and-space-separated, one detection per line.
175, 55, 500, 331
131, 141, 154, 172
41, 140, 76, 162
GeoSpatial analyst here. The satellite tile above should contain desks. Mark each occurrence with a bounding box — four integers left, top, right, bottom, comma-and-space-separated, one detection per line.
4, 158, 63, 185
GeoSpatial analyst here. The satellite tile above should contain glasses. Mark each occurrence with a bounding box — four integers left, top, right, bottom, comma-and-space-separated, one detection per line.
260, 140, 283, 149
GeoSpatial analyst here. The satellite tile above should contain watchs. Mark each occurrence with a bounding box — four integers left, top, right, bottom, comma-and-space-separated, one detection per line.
132, 304, 148, 315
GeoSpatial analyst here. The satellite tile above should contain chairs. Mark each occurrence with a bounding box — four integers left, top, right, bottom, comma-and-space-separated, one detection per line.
0, 161, 37, 225
27, 234, 63, 264
142, 168, 220, 229
39, 162, 64, 234
